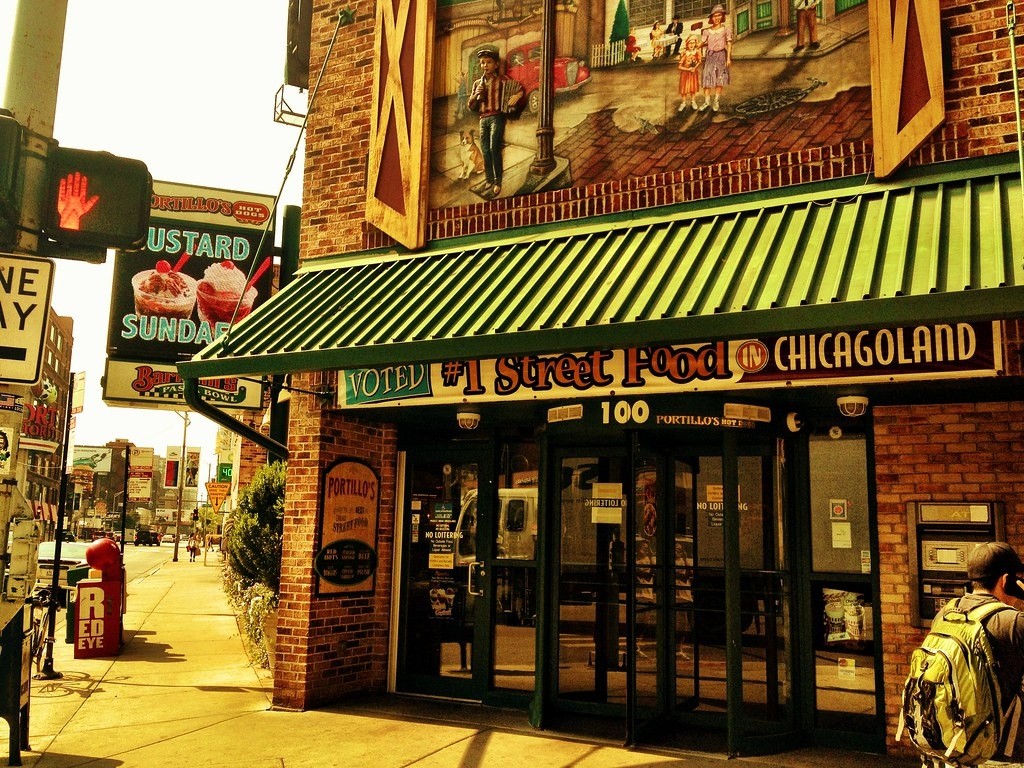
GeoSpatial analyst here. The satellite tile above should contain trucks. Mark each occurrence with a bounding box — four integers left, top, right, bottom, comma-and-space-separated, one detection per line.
124, 528, 135, 545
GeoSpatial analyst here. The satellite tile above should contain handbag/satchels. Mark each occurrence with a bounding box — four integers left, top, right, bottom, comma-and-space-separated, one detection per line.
186, 545, 190, 552
195, 547, 200, 555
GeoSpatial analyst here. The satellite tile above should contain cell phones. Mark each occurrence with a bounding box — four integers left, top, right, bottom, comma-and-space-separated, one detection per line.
1004, 576, 1024, 600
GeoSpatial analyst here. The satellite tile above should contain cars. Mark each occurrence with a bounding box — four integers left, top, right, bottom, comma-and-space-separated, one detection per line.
114, 531, 123, 541
62, 530, 76, 543
4, 540, 95, 604
105, 532, 116, 541
92, 531, 107, 541
162, 534, 173, 543
150, 531, 161, 547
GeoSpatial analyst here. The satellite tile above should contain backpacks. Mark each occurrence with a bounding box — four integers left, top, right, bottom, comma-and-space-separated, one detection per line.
896, 597, 1024, 768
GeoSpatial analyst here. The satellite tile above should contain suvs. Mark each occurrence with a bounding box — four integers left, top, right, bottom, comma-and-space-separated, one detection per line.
134, 530, 153, 547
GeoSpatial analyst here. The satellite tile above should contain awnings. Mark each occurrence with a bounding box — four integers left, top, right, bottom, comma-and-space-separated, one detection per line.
175, 162, 1024, 461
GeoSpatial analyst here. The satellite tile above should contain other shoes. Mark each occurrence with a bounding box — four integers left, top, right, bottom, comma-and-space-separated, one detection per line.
193, 560, 195, 562
190, 559, 192, 562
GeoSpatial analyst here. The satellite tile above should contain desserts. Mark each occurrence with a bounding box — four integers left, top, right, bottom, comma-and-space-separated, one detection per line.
135, 261, 196, 323
197, 261, 256, 325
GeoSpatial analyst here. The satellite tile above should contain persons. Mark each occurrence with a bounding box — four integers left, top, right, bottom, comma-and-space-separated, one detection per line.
187, 532, 197, 562
931, 543, 1024, 768
207, 531, 214, 551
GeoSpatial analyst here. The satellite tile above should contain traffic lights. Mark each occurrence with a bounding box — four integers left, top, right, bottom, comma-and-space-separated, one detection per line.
46, 147, 151, 249
192, 508, 198, 521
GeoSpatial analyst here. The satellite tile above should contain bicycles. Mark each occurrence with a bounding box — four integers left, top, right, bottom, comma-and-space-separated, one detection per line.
24, 589, 60, 672
